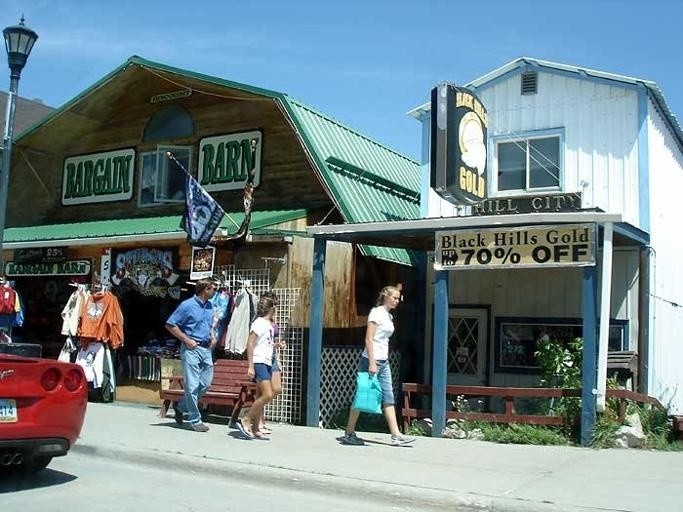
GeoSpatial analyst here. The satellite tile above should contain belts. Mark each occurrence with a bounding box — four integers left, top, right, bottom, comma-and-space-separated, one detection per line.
196, 341, 211, 347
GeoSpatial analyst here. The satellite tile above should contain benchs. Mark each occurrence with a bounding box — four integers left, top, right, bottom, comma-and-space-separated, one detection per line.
158, 358, 266, 428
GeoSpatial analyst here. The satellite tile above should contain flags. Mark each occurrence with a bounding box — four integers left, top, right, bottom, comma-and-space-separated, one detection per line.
179, 173, 225, 249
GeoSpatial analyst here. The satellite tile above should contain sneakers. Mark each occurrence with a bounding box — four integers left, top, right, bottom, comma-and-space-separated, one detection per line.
187, 422, 209, 433
342, 432, 365, 446
390, 433, 416, 446
256, 425, 271, 440
173, 401, 184, 427
234, 418, 255, 440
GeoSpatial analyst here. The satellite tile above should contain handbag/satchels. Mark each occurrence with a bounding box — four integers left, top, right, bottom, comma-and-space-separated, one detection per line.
352, 371, 383, 414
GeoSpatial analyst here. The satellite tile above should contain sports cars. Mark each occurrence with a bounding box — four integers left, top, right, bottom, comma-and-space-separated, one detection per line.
0, 349, 87, 475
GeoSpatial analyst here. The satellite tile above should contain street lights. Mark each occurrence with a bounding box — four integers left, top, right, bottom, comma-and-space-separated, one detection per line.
0, 10, 36, 253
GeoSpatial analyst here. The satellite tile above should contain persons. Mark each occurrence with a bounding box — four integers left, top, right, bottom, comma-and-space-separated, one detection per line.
341, 286, 416, 446
237, 296, 287, 440
165, 278, 217, 432
258, 293, 287, 434
